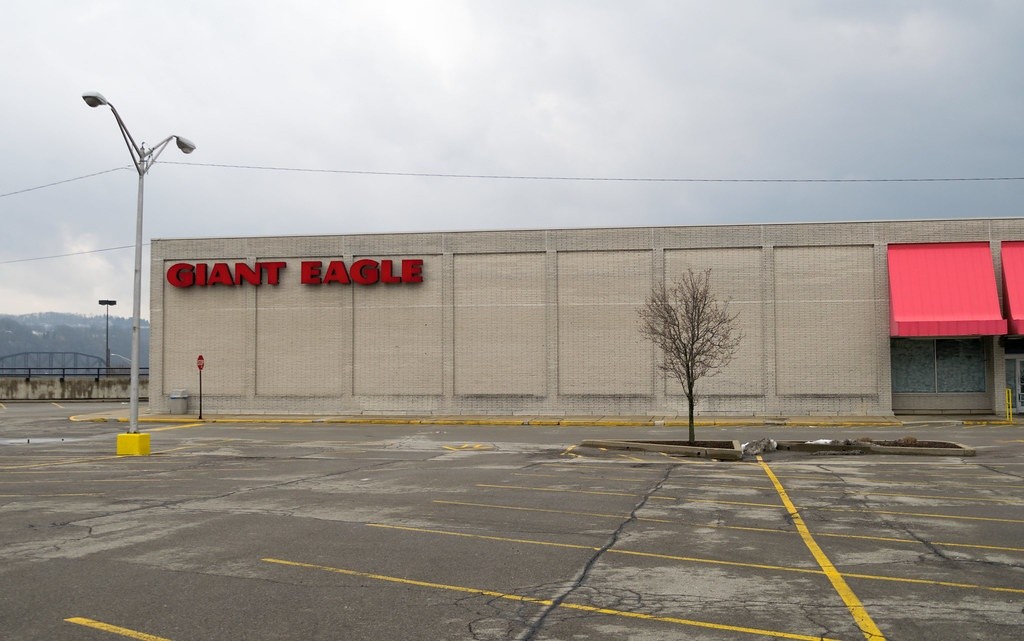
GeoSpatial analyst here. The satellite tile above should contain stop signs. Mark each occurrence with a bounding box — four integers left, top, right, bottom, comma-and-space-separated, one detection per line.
197, 355, 204, 370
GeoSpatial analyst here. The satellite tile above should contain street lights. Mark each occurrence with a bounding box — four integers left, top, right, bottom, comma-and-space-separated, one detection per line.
84, 94, 196, 434
96, 300, 118, 373
